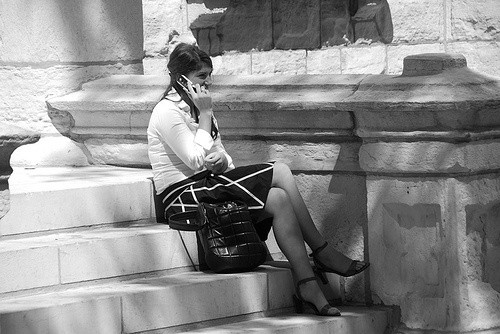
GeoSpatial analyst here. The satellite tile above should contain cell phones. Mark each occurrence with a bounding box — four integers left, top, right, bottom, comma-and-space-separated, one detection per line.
176, 75, 196, 96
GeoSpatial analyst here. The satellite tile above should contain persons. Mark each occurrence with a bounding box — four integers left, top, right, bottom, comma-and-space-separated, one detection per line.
147, 42, 370, 316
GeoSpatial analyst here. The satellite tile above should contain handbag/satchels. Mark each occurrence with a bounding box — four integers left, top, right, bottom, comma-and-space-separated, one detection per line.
169, 201, 267, 271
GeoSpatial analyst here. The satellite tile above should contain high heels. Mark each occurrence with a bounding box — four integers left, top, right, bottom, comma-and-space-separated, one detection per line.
309, 242, 369, 285
293, 277, 342, 316
